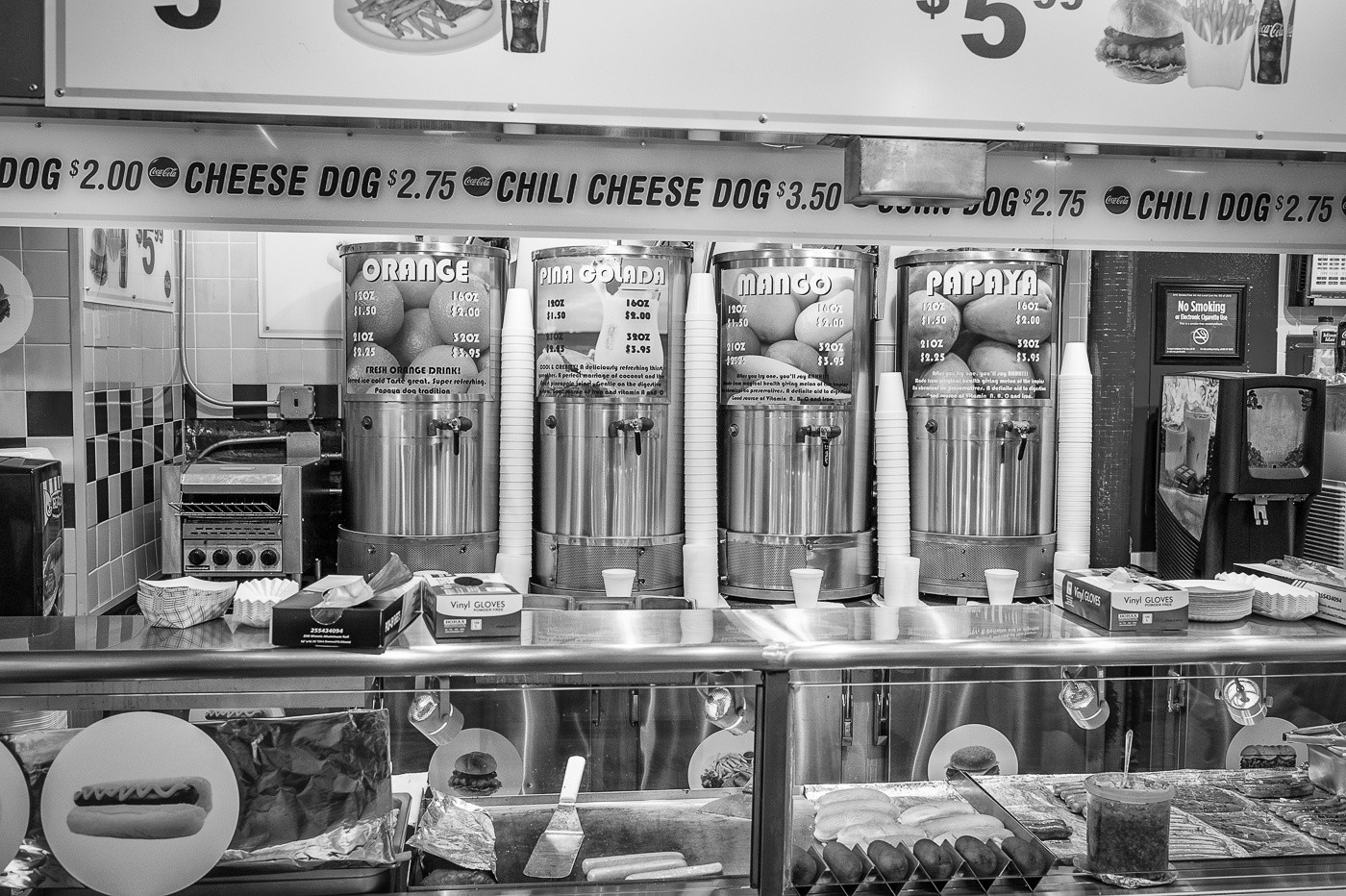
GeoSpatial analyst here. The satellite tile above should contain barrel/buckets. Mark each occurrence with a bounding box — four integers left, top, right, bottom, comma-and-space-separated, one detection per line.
532, 247, 694, 543
894, 250, 1064, 536
716, 244, 878, 535
335, 237, 508, 537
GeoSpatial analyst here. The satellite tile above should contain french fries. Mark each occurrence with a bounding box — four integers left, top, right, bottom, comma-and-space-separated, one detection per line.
1183, 0, 1259, 46
345, 0, 458, 40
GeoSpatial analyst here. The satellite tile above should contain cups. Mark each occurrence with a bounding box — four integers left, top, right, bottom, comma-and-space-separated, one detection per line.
1164, 408, 1212, 483
873, 373, 919, 610
494, 288, 533, 595
984, 569, 1018, 605
790, 568, 824, 609
601, 569, 636, 597
684, 272, 718, 610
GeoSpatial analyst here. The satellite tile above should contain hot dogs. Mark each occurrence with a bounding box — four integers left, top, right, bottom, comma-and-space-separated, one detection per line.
65, 776, 212, 839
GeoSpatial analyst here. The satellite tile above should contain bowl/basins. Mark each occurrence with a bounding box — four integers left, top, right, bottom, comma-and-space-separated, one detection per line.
137, 575, 238, 630
1307, 744, 1345, 798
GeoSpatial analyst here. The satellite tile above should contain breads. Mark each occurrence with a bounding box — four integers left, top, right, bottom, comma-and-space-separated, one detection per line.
436, 0, 494, 21
812, 788, 1018, 846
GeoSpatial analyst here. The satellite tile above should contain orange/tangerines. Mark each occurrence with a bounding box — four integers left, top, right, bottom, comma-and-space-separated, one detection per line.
344, 252, 497, 396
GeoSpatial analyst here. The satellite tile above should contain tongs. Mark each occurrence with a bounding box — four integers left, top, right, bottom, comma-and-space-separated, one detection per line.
1283, 720, 1346, 758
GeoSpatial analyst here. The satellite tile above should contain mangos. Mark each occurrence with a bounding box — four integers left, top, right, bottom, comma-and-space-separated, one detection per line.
719, 268, 1054, 398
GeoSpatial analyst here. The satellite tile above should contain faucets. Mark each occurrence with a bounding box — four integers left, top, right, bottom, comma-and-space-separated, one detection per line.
426, 416, 472, 456
608, 416, 654, 456
996, 419, 1038, 460
795, 424, 841, 467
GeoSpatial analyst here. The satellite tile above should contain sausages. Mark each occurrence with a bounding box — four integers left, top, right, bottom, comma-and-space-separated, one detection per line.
582, 850, 723, 881
791, 835, 1044, 884
1009, 780, 1233, 861
1234, 774, 1346, 850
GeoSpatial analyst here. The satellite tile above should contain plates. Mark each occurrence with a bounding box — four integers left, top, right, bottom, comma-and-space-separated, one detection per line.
0, 711, 240, 895
428, 728, 523, 800
234, 577, 300, 628
688, 730, 754, 788
928, 724, 1019, 777
1215, 572, 1318, 621
1225, 718, 1309, 770
1167, 579, 1255, 622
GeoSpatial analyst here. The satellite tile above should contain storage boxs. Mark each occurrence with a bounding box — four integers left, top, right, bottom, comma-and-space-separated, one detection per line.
137, 576, 238, 629
1233, 563, 1346, 626
1053, 568, 1189, 631
271, 575, 423, 652
423, 569, 523, 638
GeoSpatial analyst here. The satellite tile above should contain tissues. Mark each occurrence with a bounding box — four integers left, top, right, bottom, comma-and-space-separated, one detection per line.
268, 551, 422, 648
1053, 566, 1189, 634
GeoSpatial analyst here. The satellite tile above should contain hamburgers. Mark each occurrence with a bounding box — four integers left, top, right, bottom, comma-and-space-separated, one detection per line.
1093, 0, 1189, 85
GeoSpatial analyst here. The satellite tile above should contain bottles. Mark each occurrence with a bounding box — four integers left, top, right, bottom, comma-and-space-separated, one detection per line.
1312, 312, 1346, 385
1083, 774, 1173, 880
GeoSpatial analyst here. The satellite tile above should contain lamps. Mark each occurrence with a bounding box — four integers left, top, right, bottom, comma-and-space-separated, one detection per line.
407, 677, 463, 748
694, 674, 755, 736
1057, 667, 1110, 730
1214, 665, 1273, 726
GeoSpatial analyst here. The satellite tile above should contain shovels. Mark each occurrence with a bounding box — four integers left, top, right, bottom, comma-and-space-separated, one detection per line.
522, 754, 585, 879
